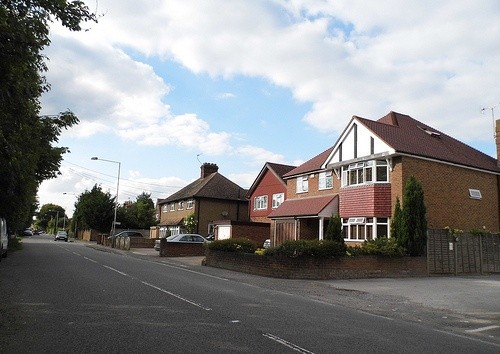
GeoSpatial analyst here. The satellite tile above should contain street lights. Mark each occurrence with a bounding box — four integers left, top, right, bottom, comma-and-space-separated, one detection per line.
91, 157, 121, 249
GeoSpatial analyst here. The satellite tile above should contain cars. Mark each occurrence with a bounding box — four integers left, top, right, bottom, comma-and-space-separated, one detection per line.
55, 231, 69, 242
205, 233, 215, 240
24, 228, 46, 237
154, 234, 212, 251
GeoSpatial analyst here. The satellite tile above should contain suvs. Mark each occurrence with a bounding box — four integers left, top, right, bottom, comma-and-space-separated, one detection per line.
108, 231, 143, 243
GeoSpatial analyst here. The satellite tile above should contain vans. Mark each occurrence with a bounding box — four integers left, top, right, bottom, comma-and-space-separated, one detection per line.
0, 218, 9, 257
